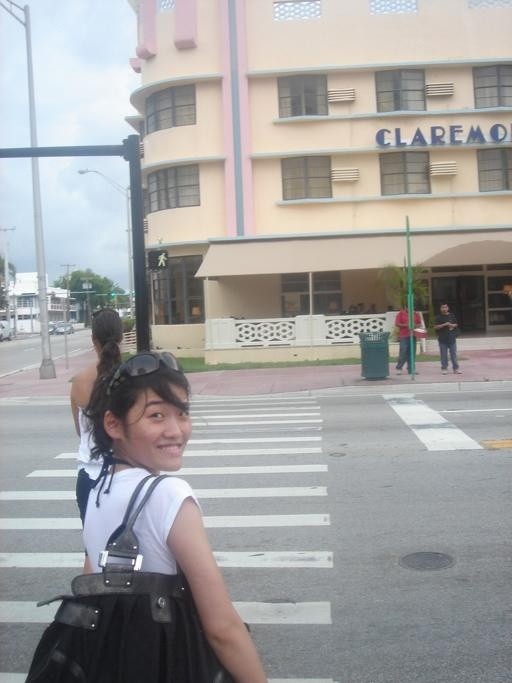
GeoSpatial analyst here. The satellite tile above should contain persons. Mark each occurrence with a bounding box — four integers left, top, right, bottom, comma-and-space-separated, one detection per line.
394, 302, 463, 375
82, 351, 266, 683
69, 309, 124, 574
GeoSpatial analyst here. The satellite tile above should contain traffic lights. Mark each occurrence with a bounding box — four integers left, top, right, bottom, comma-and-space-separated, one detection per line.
108, 293, 117, 296
149, 251, 167, 270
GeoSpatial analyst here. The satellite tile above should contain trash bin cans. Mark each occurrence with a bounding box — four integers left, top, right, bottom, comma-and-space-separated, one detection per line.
359, 331, 391, 381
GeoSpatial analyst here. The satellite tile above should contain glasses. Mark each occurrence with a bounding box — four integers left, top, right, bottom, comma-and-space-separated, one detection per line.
105, 352, 184, 399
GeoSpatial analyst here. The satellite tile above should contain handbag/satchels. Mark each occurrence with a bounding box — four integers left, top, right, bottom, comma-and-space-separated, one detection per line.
25, 475, 250, 683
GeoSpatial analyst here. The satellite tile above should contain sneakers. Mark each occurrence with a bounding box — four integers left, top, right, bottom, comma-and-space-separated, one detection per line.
442, 368, 463, 375
396, 368, 420, 375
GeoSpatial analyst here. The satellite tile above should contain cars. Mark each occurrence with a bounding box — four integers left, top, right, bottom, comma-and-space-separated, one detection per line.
0, 321, 13, 341
49, 322, 74, 335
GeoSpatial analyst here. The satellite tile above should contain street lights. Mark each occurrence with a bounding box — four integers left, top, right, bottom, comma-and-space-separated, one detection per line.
77, 170, 134, 319
